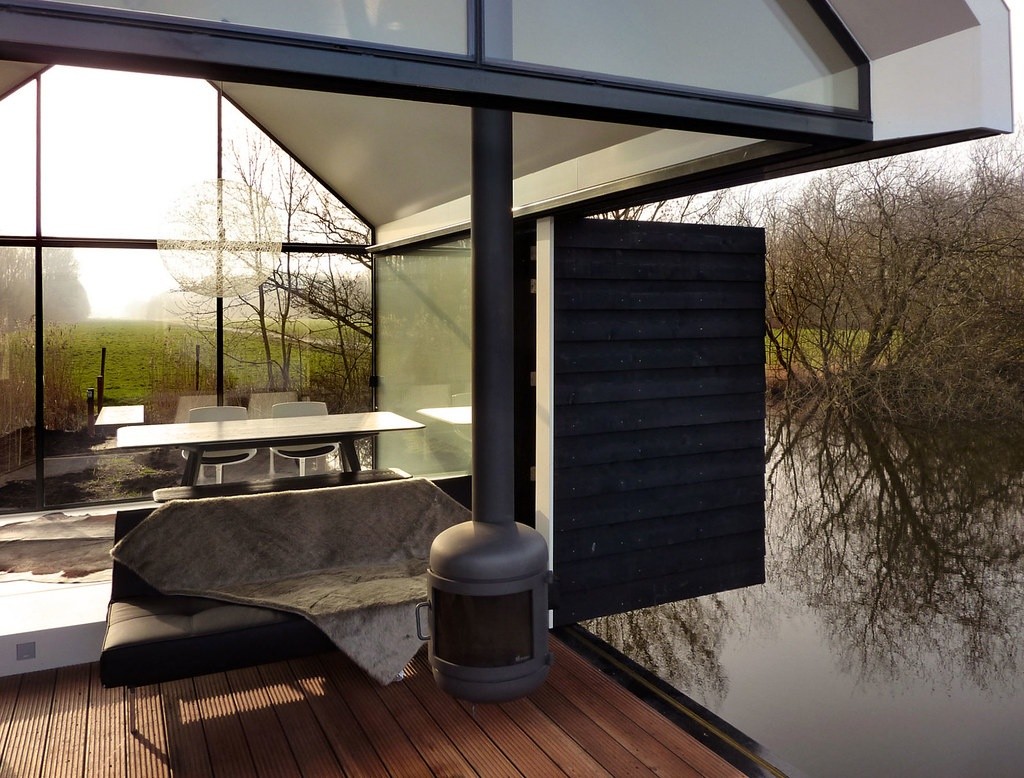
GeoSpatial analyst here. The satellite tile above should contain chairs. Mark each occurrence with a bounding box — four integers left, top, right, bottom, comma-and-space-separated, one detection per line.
268, 402, 339, 476
182, 406, 258, 484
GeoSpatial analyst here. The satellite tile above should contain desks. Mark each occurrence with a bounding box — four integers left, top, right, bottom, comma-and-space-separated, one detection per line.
116, 411, 426, 487
94, 404, 146, 426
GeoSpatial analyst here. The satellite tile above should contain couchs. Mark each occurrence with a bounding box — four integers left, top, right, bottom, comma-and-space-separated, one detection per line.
97, 478, 473, 734
